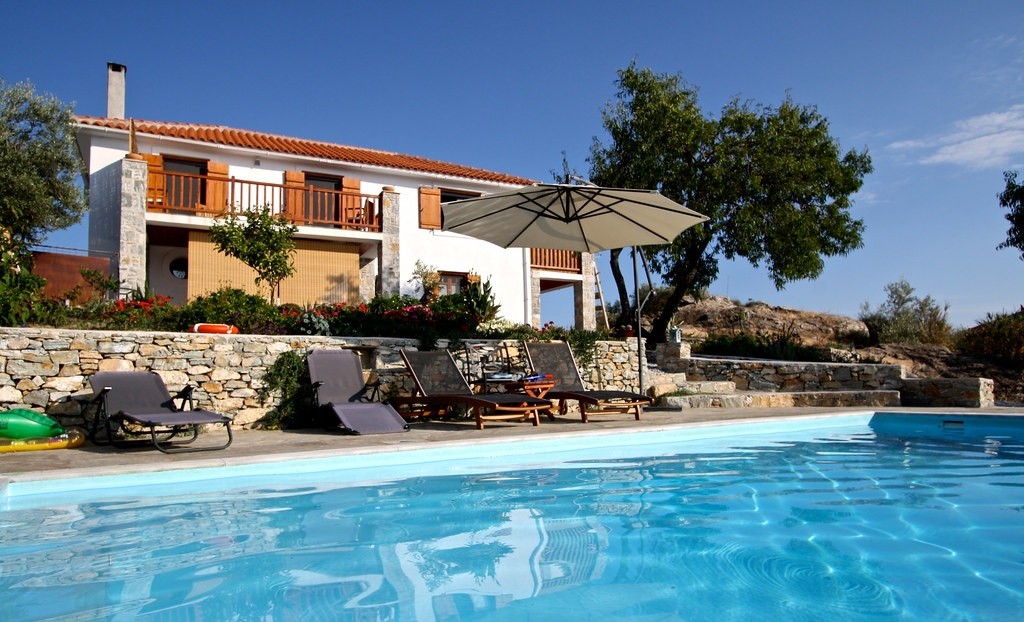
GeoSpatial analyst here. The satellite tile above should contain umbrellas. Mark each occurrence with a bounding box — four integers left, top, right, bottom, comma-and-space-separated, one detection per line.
440, 172, 710, 413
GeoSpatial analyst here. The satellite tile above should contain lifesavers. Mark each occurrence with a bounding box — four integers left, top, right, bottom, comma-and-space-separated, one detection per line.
187, 322, 239, 333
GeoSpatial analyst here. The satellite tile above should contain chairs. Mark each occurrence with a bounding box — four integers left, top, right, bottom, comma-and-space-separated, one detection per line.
523, 340, 653, 423
345, 200, 374, 231
398, 348, 553, 430
306, 350, 411, 435
74, 371, 232, 454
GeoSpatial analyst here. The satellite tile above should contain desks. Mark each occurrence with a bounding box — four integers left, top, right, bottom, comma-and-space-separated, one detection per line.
485, 380, 556, 422
